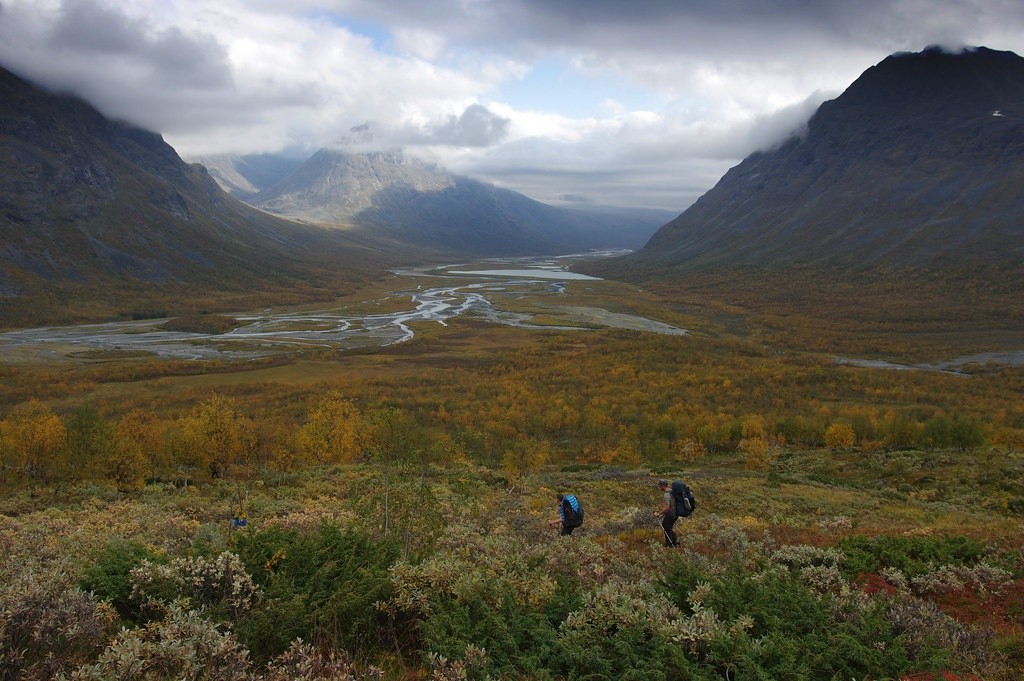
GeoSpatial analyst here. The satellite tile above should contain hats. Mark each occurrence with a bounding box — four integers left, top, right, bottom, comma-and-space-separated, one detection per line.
658, 479, 667, 485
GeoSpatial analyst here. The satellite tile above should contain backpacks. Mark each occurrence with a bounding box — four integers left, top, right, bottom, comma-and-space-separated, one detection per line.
563, 494, 583, 528
665, 480, 696, 517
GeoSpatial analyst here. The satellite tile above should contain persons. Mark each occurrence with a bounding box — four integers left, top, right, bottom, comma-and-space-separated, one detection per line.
547, 494, 584, 537
653, 478, 696, 548
